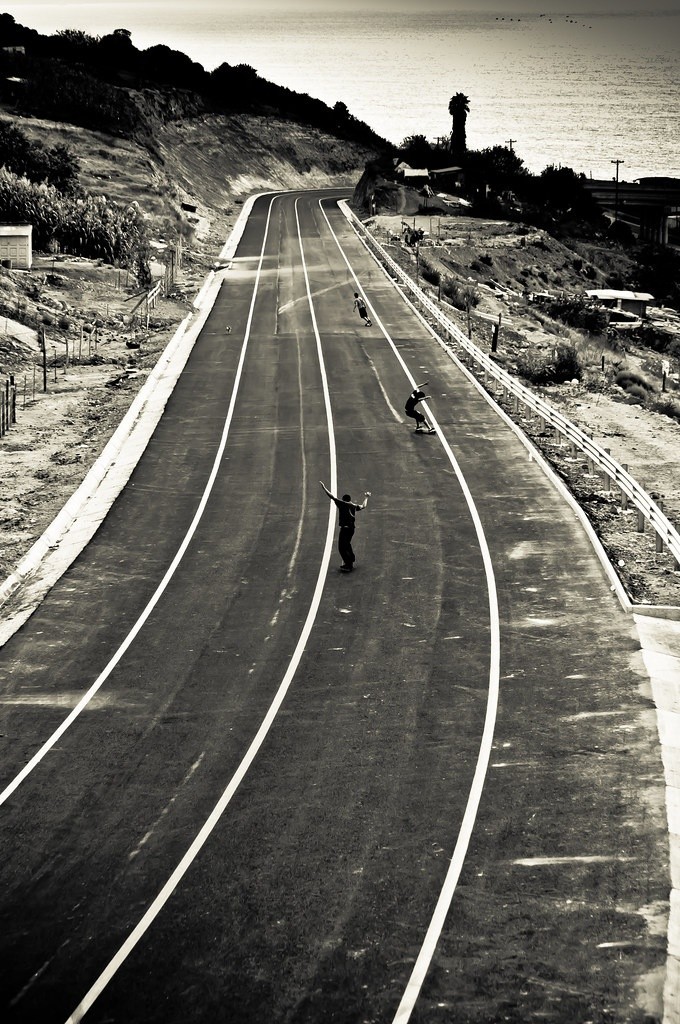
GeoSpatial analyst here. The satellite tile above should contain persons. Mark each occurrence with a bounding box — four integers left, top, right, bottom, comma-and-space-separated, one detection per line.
353, 293, 372, 326
404, 380, 436, 434
319, 481, 372, 573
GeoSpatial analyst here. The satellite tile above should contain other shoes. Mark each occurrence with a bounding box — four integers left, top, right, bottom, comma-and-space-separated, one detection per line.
429, 428, 434, 432
416, 426, 423, 430
365, 322, 372, 327
340, 565, 350, 571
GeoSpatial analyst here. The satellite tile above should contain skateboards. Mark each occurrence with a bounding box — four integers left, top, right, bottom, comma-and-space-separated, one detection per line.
414, 426, 436, 434
341, 560, 353, 572
365, 322, 372, 327
